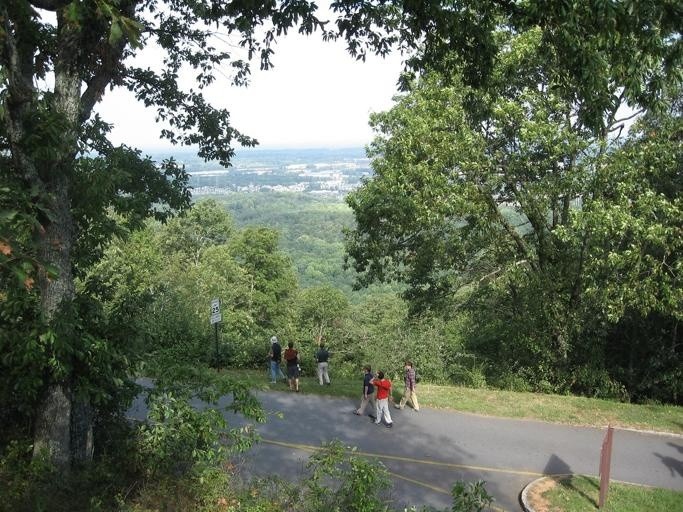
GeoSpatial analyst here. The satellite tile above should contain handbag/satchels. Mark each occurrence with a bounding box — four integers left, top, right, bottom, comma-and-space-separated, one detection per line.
404, 374, 422, 385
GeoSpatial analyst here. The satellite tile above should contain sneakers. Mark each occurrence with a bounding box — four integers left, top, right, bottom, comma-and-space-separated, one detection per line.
354, 403, 393, 429
395, 404, 420, 412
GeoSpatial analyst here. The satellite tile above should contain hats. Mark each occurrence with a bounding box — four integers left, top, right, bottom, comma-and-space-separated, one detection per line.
271, 336, 278, 343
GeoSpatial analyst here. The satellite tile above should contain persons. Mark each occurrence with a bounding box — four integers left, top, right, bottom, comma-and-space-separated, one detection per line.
315, 343, 331, 386
395, 361, 419, 411
269, 336, 301, 392
353, 365, 393, 428
